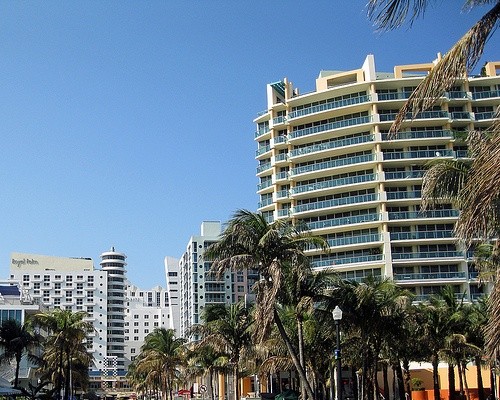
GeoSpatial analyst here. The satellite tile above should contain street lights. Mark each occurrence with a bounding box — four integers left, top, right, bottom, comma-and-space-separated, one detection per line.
332, 306, 343, 400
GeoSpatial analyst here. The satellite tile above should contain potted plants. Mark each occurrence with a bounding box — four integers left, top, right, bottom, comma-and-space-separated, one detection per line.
409, 376, 428, 400
